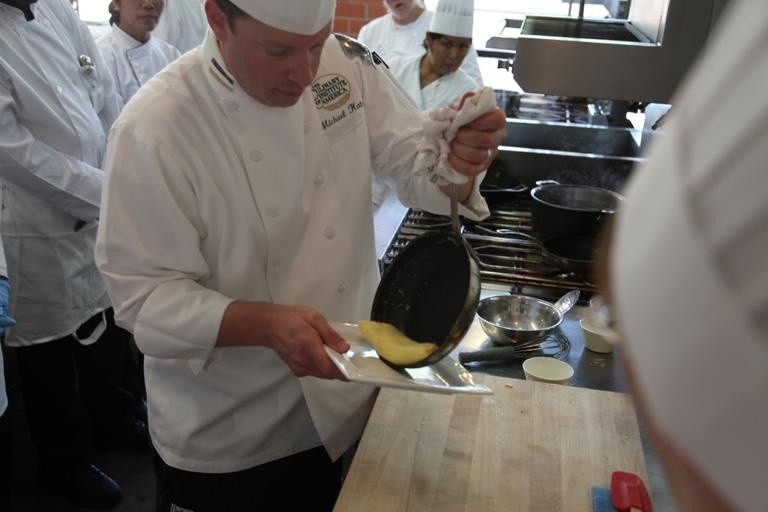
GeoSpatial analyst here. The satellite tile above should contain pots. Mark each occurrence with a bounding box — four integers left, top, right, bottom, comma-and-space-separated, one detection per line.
367, 92, 482, 372
529, 179, 631, 262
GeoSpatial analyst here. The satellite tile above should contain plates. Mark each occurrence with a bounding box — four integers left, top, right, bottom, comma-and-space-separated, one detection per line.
317, 320, 496, 397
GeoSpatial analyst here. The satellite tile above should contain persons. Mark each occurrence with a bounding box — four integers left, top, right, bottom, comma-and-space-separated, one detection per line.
95, 0, 509, 511
1, 0, 209, 512
356, 1, 484, 212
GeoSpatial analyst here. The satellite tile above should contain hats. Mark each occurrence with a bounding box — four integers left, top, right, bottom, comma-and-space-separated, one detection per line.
429, 0, 474, 39
608, 0, 768, 512
228, 0, 337, 36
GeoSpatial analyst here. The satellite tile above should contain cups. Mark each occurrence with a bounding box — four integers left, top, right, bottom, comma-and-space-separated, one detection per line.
579, 319, 619, 354
521, 354, 575, 386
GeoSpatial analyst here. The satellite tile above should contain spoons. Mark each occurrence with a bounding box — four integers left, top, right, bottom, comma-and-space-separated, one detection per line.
552, 289, 580, 317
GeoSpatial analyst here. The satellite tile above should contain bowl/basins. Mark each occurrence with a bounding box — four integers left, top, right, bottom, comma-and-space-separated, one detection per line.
469, 293, 565, 346
